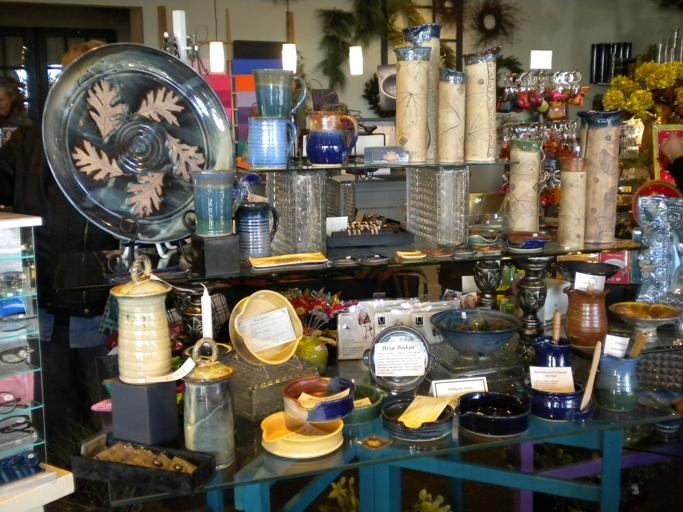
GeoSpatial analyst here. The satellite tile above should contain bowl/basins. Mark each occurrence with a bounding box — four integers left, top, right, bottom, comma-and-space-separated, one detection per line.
429, 307, 522, 361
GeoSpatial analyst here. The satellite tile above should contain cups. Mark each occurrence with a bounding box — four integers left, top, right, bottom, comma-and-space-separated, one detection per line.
305, 111, 359, 166
253, 68, 308, 117
188, 169, 244, 237
235, 201, 280, 261
246, 117, 297, 170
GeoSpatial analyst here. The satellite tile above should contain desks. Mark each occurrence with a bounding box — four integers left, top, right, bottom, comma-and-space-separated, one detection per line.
78, 152, 683, 512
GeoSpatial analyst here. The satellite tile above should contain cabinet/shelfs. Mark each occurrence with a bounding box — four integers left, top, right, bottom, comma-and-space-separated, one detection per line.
1, 210, 77, 512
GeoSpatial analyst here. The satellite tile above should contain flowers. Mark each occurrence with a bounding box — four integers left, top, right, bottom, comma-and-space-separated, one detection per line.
600, 58, 683, 122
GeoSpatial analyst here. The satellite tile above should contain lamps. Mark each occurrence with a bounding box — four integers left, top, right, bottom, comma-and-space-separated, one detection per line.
347, 1, 364, 76
205, 1, 228, 76
280, 0, 298, 73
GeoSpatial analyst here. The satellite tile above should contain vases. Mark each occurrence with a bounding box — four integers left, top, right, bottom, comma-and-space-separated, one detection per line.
639, 106, 676, 163
398, 21, 629, 252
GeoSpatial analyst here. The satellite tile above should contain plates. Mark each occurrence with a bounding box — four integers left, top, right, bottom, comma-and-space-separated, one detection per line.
41, 42, 236, 243
247, 250, 329, 267
363, 324, 438, 394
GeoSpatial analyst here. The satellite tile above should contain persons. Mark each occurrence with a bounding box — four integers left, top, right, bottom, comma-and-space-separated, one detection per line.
662, 132, 683, 193
0, 78, 35, 147
0, 38, 118, 428
